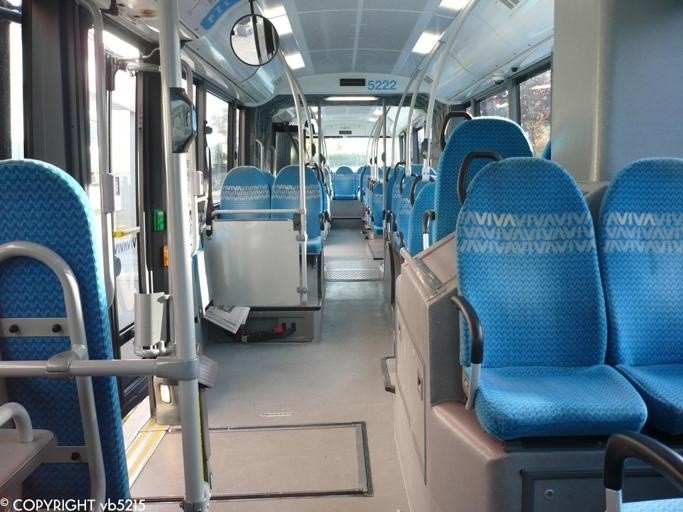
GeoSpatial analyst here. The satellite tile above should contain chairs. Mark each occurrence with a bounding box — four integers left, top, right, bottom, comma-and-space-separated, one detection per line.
220, 165, 332, 255
360, 164, 436, 258
331, 166, 362, 200
435, 115, 683, 442
